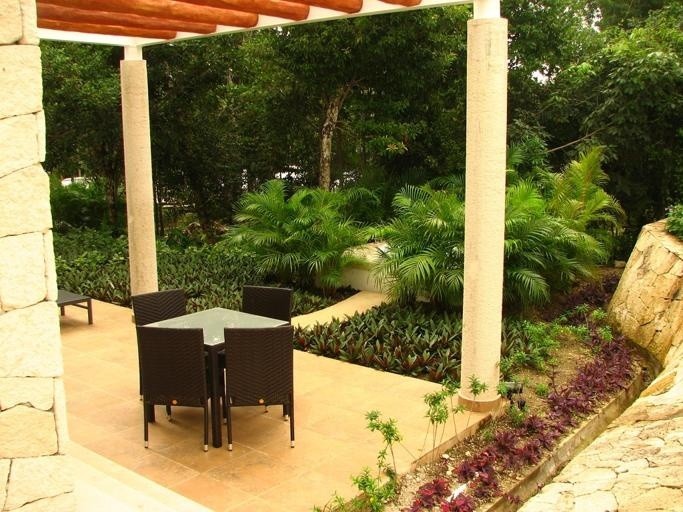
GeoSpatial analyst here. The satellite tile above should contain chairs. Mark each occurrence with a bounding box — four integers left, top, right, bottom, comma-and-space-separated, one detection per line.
131, 286, 294, 452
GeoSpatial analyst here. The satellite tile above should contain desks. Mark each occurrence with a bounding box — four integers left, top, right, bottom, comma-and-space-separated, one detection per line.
58, 290, 92, 324
